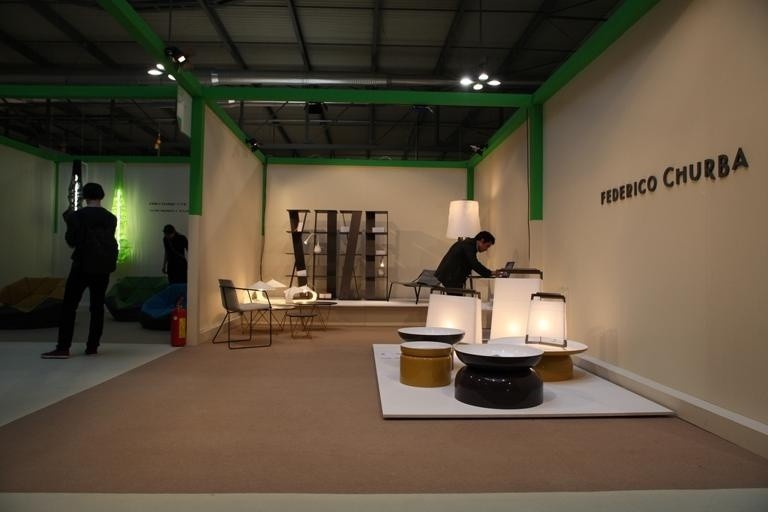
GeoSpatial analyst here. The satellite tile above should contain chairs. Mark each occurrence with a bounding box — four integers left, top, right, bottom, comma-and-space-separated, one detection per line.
388, 268, 445, 305
209, 279, 274, 350
0, 274, 187, 332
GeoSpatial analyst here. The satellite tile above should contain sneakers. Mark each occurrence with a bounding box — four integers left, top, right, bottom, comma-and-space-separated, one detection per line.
84, 342, 100, 357
40, 344, 71, 359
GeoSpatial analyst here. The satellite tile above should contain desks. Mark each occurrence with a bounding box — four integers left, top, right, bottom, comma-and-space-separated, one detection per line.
238, 302, 295, 336
291, 300, 337, 329
465, 271, 544, 303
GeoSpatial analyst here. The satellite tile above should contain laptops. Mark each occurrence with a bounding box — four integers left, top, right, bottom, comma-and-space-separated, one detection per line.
491, 262, 515, 278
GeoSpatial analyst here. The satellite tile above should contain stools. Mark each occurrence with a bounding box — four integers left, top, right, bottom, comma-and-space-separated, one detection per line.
286, 311, 318, 340
392, 325, 587, 411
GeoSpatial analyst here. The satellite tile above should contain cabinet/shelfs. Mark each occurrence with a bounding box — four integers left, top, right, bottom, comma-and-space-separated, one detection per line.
281, 207, 389, 300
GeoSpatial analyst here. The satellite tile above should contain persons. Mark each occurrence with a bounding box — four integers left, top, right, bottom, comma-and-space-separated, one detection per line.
40, 182, 117, 359
162, 224, 188, 283
433, 230, 506, 296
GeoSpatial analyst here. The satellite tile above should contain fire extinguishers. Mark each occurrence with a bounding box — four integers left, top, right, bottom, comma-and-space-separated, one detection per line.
170, 299, 185, 345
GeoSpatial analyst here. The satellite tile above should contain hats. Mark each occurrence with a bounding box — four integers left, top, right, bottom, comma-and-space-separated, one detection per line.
82, 182, 105, 199
162, 224, 175, 233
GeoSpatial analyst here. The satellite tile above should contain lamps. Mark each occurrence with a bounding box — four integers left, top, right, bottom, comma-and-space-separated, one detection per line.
245, 136, 262, 154
246, 281, 273, 301
162, 44, 190, 71
468, 143, 488, 157
422, 287, 483, 367
302, 99, 329, 115
445, 200, 482, 243
458, 0, 503, 92
522, 292, 568, 349
263, 278, 287, 291
487, 267, 544, 341
